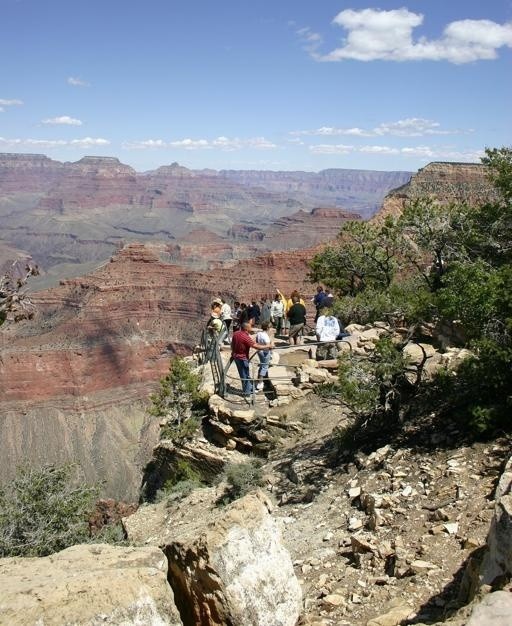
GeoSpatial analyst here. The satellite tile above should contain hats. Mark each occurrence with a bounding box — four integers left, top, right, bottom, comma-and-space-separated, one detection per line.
211, 312, 221, 319
259, 297, 267, 305
251, 298, 256, 303
213, 298, 223, 305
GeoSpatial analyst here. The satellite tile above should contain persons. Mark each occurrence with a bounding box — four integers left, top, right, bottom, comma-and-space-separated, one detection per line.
310, 285, 326, 322
255, 320, 273, 391
207, 287, 306, 348
230, 322, 274, 396
323, 289, 333, 298
315, 307, 339, 373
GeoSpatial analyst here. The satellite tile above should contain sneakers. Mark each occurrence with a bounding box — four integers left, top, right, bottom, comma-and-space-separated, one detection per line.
256, 381, 265, 390
